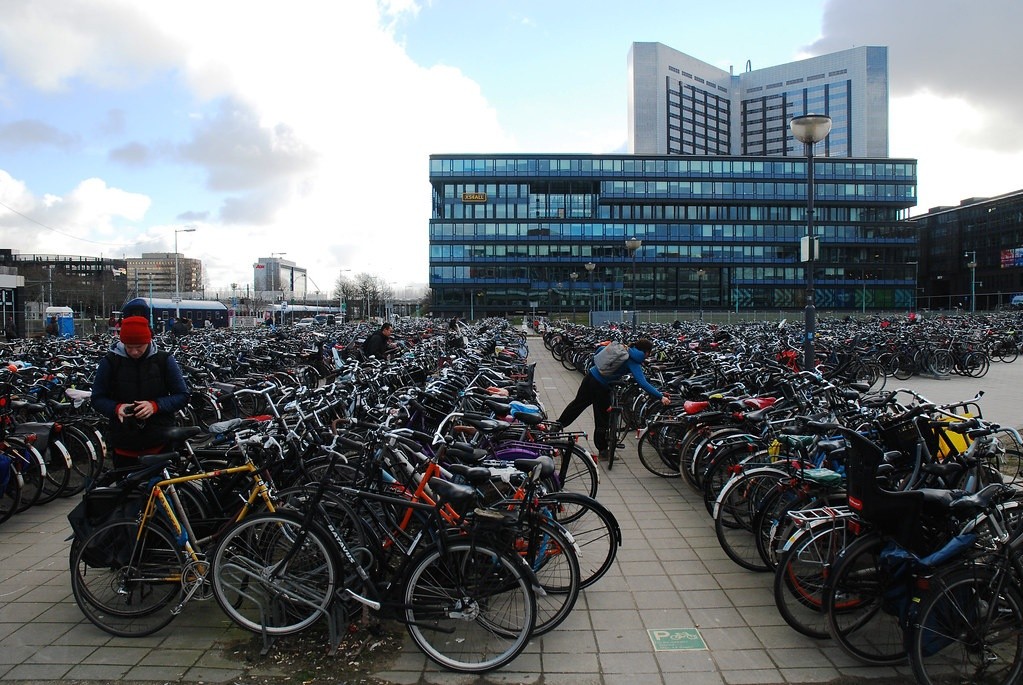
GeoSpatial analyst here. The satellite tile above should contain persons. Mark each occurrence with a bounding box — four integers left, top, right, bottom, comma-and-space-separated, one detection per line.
204, 316, 212, 329
92, 316, 191, 483
157, 315, 193, 337
45, 317, 59, 337
107, 314, 122, 335
4, 317, 17, 342
266, 316, 274, 327
442, 316, 458, 330
363, 322, 397, 361
548, 340, 670, 461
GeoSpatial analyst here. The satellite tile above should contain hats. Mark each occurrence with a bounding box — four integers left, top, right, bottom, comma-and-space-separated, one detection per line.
120, 317, 151, 344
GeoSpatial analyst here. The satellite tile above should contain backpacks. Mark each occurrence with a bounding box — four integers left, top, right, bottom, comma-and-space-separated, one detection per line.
594, 341, 630, 377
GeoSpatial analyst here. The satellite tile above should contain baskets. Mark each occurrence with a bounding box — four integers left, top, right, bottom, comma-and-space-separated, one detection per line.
880, 415, 938, 469
419, 398, 455, 438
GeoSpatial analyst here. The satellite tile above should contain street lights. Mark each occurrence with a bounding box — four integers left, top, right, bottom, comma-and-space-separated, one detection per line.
405, 286, 413, 316
964, 249, 976, 313
570, 272, 579, 319
584, 261, 596, 328
231, 283, 238, 327
175, 228, 196, 318
789, 113, 832, 373
556, 283, 564, 321
314, 290, 320, 314
368, 277, 377, 321
339, 269, 351, 314
388, 281, 397, 320
696, 270, 705, 326
271, 252, 287, 326
625, 236, 642, 330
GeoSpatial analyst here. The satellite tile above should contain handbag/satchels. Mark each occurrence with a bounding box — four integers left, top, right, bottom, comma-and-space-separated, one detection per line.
67, 480, 173, 569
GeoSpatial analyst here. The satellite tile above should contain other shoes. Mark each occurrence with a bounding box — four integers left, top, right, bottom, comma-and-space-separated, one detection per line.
599, 449, 620, 461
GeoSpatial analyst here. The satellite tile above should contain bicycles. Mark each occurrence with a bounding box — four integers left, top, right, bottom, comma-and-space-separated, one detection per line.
1, 307, 1023, 685
